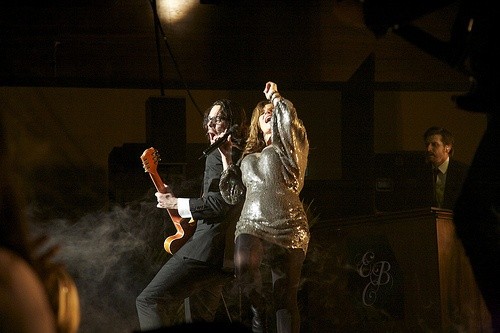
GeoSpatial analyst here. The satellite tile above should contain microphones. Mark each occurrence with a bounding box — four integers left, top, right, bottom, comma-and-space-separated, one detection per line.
198, 124, 239, 159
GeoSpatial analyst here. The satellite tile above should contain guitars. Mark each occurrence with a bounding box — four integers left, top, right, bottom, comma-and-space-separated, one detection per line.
140, 147, 197, 256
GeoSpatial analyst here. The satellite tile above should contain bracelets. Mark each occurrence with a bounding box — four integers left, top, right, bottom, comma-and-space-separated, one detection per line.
269, 90, 278, 99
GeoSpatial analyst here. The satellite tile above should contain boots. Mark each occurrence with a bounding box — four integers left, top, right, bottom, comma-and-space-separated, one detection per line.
249, 303, 267, 333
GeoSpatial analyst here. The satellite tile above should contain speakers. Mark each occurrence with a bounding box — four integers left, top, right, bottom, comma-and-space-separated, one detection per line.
144, 97, 187, 164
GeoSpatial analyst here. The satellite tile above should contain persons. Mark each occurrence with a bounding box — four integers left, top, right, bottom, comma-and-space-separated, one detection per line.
135, 97, 247, 333
211, 82, 309, 333
0, 134, 80, 333
411, 126, 468, 211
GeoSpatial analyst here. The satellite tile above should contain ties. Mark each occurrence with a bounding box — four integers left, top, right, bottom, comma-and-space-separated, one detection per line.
433, 167, 441, 186
